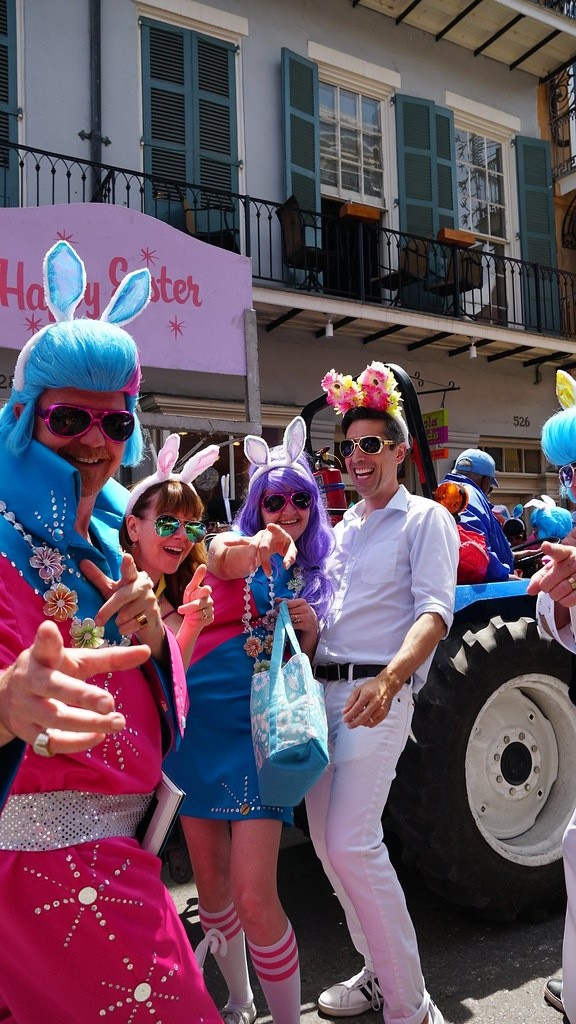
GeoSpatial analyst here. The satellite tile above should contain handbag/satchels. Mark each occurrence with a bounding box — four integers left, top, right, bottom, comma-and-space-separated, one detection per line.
251, 600, 328, 807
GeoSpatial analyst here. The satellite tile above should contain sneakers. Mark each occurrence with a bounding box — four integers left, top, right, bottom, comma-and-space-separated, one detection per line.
317, 962, 386, 1016
220, 1001, 257, 1024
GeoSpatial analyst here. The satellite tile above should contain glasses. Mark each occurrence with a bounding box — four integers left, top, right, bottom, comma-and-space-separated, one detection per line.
259, 490, 313, 514
558, 460, 576, 488
339, 435, 395, 459
137, 515, 207, 543
34, 402, 136, 443
507, 532, 524, 542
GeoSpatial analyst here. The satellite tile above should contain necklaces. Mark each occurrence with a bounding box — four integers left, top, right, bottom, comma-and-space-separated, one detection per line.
0, 500, 132, 649
242, 562, 306, 674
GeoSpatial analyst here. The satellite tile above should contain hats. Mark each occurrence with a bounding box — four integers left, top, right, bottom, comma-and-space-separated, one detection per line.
454, 446, 499, 489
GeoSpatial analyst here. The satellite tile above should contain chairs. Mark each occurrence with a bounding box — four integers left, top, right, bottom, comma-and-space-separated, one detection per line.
435, 241, 483, 323
180, 198, 237, 254
275, 196, 328, 292
379, 233, 434, 307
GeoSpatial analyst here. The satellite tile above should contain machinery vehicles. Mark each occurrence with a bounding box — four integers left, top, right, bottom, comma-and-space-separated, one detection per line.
293, 363, 575, 935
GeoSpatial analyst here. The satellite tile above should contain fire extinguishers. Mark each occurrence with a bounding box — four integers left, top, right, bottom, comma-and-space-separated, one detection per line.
313, 445, 347, 527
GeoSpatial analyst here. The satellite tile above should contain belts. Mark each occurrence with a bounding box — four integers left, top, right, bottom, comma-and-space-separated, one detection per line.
309, 661, 412, 685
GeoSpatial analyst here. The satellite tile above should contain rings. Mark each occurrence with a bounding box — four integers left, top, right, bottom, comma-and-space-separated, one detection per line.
567, 576, 576, 590
201, 608, 207, 620
33, 727, 55, 756
135, 613, 148, 630
369, 716, 375, 723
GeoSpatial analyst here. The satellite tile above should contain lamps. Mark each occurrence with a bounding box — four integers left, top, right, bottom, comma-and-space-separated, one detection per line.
468, 337, 479, 358
325, 314, 337, 338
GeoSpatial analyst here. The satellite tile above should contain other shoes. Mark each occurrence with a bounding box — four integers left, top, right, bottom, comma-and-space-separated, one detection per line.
546, 978, 565, 1011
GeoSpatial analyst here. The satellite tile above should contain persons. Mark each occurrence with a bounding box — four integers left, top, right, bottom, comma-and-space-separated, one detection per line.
435, 368, 576, 1024
0, 240, 337, 1024
303, 360, 459, 1024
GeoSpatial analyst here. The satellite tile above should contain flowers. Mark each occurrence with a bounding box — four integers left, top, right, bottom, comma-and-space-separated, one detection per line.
320, 360, 405, 416
